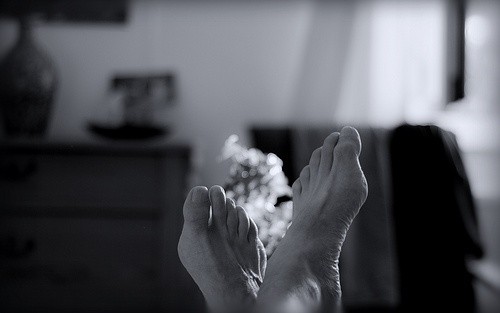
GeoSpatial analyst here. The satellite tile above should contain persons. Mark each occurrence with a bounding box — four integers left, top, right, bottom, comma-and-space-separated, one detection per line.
179, 125, 370, 313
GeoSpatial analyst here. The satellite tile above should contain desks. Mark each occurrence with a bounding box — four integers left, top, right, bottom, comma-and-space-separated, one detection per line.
0, 132, 193, 313
